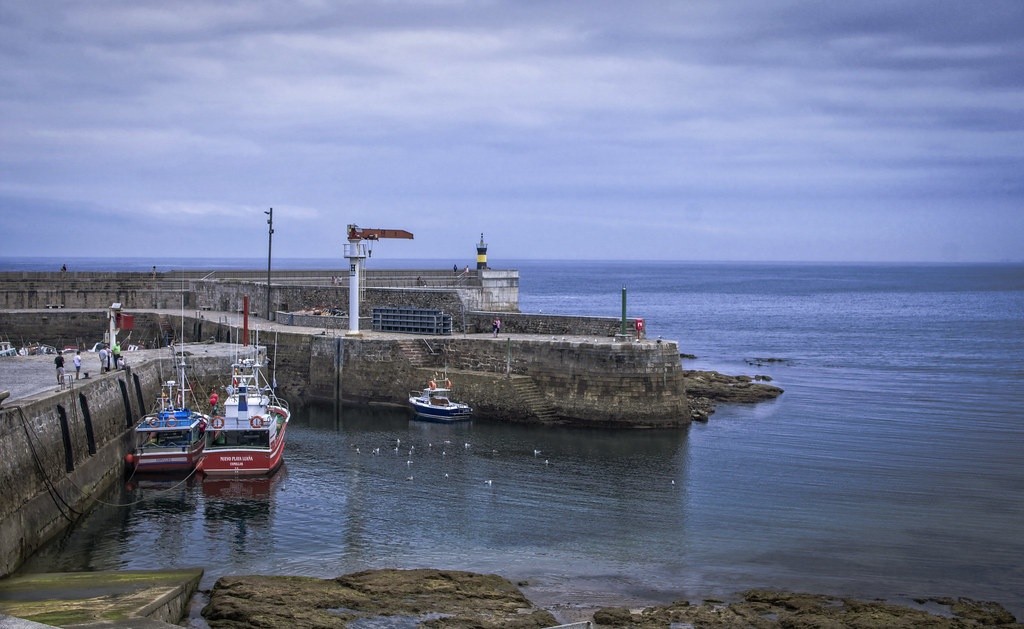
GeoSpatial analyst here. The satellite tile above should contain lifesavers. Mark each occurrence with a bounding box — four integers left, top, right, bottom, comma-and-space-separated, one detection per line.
166, 417, 177, 427
211, 416, 225, 429
149, 417, 160, 428
429, 381, 434, 390
249, 416, 263, 428
445, 380, 451, 388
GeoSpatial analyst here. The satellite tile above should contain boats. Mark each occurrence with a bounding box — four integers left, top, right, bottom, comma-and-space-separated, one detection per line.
198, 324, 290, 479
0, 337, 144, 351
129, 292, 215, 472
409, 377, 472, 421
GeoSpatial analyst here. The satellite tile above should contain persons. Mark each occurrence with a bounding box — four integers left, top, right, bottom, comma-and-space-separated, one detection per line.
99, 345, 108, 374
111, 340, 121, 369
417, 276, 424, 286
453, 264, 457, 272
53, 350, 65, 385
104, 343, 112, 372
60, 264, 67, 272
492, 317, 500, 338
72, 350, 82, 380
492, 319, 498, 338
330, 275, 343, 286
